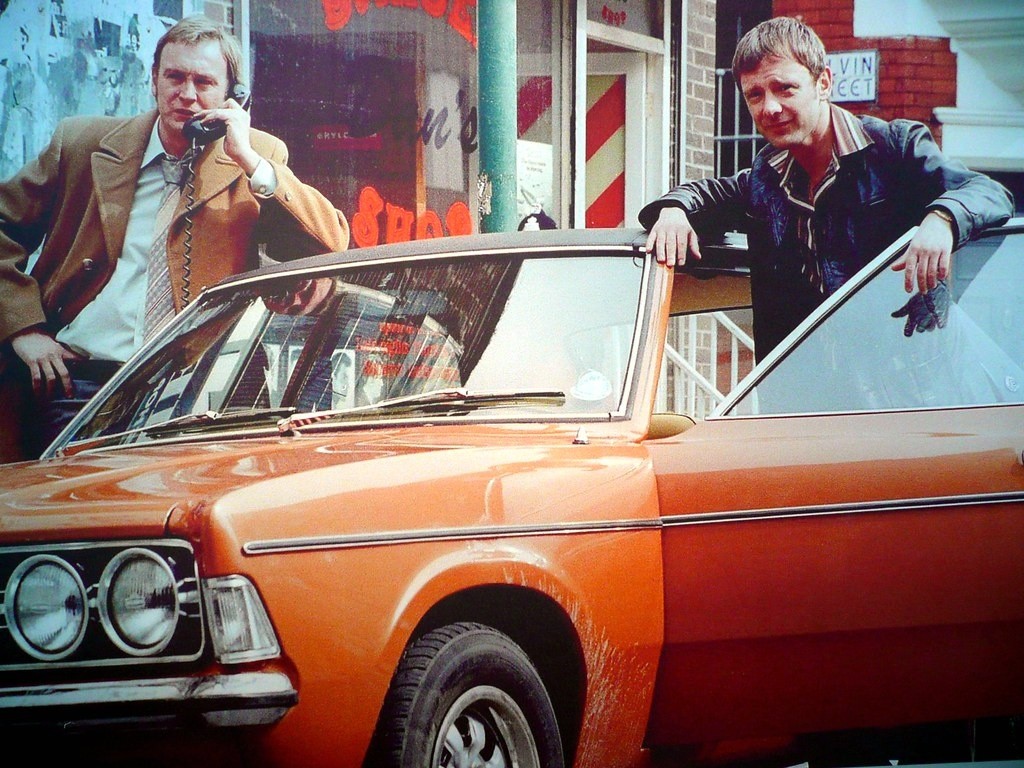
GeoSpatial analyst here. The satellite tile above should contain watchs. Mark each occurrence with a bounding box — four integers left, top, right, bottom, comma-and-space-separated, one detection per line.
929, 208, 957, 225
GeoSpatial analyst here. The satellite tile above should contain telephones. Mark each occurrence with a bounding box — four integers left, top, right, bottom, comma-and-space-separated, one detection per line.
186, 82, 253, 143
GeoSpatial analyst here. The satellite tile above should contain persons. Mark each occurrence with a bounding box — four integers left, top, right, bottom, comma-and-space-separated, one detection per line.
265, 278, 337, 318
634, 16, 1015, 414
1, 16, 354, 467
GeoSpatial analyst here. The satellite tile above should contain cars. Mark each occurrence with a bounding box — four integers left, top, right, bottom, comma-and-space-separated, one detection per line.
0, 229, 1024, 768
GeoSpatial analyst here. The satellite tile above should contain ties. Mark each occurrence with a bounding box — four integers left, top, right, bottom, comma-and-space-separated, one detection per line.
144, 156, 188, 349
